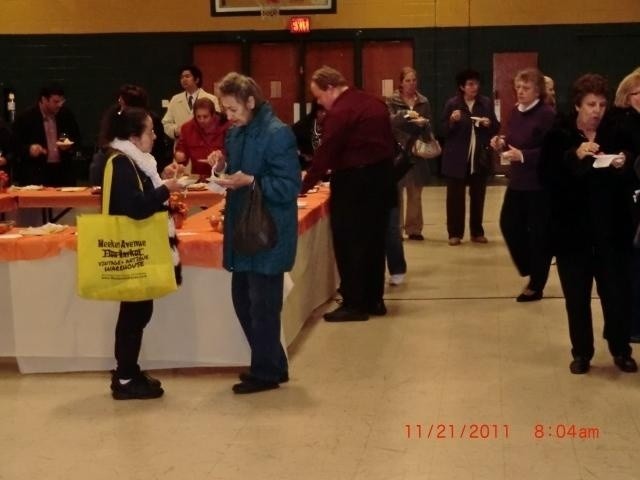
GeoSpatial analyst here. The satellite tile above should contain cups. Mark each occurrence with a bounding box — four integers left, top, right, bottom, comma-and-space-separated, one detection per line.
500, 154, 511, 165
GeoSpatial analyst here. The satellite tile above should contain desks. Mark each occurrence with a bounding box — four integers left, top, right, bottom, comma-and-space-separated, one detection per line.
0, 180, 342, 375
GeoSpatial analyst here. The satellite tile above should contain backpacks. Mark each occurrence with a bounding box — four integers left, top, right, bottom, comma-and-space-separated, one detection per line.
388, 135, 414, 182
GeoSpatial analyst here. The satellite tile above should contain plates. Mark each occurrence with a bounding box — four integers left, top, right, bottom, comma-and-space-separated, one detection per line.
592, 153, 625, 162
205, 177, 236, 182
56, 142, 75, 147
168, 178, 198, 186
296, 201, 310, 208
470, 116, 490, 123
408, 118, 432, 124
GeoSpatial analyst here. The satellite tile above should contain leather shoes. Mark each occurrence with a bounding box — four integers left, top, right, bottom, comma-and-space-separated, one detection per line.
324, 298, 387, 322
232, 367, 289, 395
613, 355, 637, 372
449, 235, 488, 245
516, 286, 543, 302
569, 357, 590, 374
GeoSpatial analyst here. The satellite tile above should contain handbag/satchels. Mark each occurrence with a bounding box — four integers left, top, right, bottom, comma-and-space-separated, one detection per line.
74, 152, 179, 301
229, 181, 278, 254
409, 121, 443, 159
475, 127, 497, 182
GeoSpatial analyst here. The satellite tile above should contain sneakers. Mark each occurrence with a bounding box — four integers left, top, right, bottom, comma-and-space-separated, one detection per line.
389, 272, 405, 286
408, 232, 424, 241
602, 329, 640, 344
110, 372, 165, 400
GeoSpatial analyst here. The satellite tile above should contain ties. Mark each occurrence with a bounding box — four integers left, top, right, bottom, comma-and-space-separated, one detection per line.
187, 96, 193, 111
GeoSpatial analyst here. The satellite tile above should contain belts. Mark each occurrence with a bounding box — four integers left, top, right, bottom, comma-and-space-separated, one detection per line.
330, 159, 389, 176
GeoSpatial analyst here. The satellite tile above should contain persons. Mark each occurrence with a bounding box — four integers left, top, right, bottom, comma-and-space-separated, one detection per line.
88, 85, 175, 184
488, 68, 558, 304
207, 72, 302, 395
540, 72, 639, 374
612, 68, 640, 111
300, 65, 396, 323
386, 64, 433, 241
435, 72, 498, 247
89, 107, 188, 398
5, 83, 85, 188
173, 96, 228, 180
158, 66, 222, 140
291, 98, 331, 184
